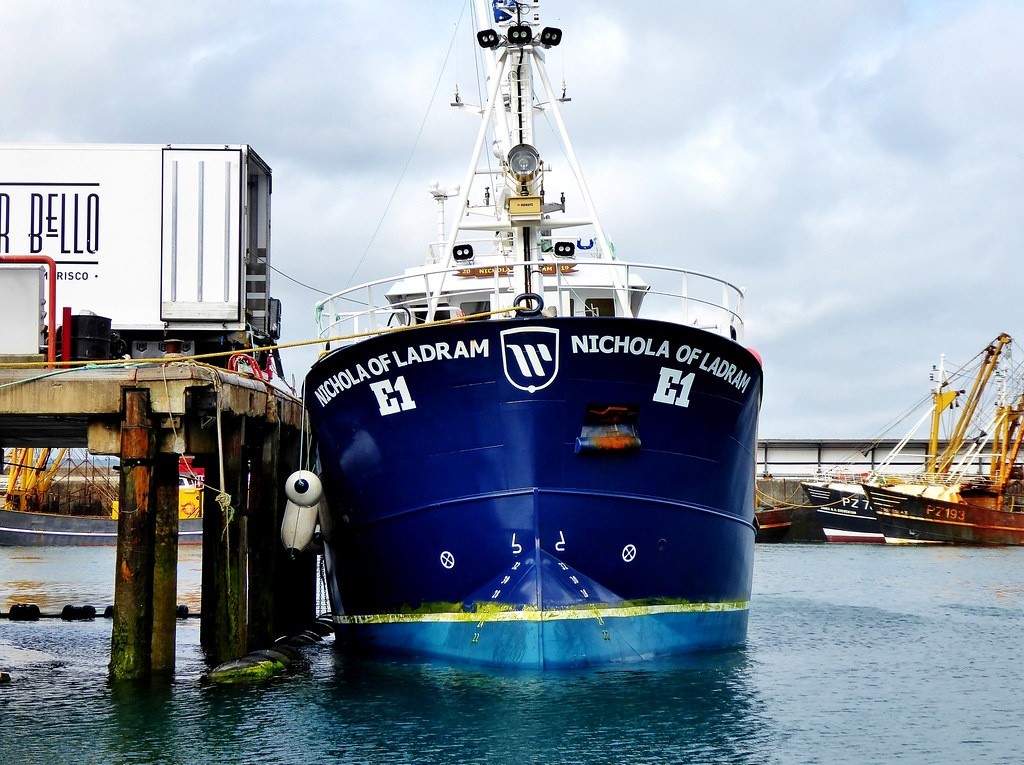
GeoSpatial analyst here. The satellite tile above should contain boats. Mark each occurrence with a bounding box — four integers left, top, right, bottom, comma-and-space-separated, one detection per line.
299, 0, 768, 678
0, 438, 217, 547
796, 350, 985, 544
752, 499, 796, 542
867, 334, 1024, 550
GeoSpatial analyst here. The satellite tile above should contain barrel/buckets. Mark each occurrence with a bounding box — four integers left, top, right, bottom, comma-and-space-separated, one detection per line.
69, 315, 112, 368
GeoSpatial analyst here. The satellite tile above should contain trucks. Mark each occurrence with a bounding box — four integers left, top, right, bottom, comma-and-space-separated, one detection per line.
0, 139, 291, 373
